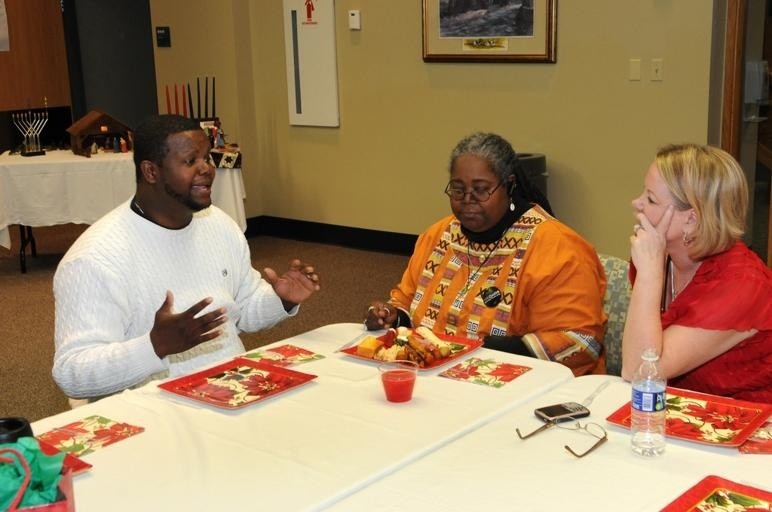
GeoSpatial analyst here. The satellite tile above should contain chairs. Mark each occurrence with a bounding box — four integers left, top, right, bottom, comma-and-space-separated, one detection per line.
598, 254, 631, 373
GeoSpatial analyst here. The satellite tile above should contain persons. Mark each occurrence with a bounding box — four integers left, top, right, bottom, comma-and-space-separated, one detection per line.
623, 145, 772, 405
363, 131, 608, 377
52, 114, 321, 403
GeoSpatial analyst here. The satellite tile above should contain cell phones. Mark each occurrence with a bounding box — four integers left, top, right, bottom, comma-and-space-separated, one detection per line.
533, 401, 590, 424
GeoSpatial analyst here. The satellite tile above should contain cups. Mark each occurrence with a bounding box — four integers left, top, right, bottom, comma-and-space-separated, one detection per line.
377, 360, 418, 403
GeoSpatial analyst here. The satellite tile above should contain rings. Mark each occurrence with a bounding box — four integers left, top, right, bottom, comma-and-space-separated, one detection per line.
367, 305, 374, 312
364, 320, 367, 324
383, 308, 392, 317
634, 227, 640, 235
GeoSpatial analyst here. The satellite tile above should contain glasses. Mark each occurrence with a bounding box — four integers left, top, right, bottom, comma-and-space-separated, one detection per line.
445, 176, 503, 201
515, 415, 608, 459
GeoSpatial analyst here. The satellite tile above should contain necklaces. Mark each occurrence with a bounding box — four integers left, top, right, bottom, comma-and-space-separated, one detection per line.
669, 259, 702, 301
133, 199, 150, 216
461, 226, 510, 295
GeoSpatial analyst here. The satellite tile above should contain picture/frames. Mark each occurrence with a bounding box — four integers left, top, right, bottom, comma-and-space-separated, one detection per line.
421, 0, 559, 65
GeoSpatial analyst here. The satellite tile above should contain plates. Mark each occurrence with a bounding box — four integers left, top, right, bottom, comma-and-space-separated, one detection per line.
658, 474, 772, 512
157, 357, 318, 410
606, 386, 772, 447
334, 333, 483, 375
37, 438, 92, 476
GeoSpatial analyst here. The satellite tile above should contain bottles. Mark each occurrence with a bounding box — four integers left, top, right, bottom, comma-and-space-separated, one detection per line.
631, 347, 667, 457
